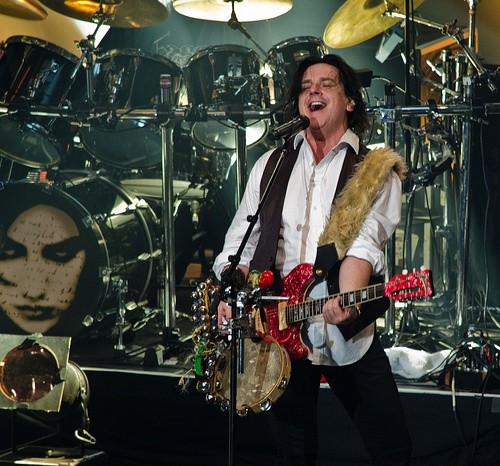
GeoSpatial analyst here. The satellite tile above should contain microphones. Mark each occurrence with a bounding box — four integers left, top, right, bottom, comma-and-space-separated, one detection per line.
268, 114, 311, 141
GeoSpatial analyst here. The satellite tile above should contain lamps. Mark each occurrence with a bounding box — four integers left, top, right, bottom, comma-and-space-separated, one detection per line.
0, 329, 99, 460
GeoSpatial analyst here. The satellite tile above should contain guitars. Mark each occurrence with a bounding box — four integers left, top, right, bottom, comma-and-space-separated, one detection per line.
246, 263, 436, 360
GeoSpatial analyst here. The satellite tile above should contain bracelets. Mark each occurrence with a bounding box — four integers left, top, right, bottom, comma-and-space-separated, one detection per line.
356, 304, 363, 316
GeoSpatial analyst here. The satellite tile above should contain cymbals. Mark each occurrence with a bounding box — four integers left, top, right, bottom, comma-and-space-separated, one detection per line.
0, 0, 48, 20
323, 0, 425, 49
190, 280, 288, 417
171, 0, 294, 22
38, 0, 168, 28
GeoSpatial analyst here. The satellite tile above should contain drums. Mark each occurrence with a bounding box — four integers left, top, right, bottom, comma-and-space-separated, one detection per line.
0, 34, 84, 169
263, 35, 328, 124
0, 169, 163, 351
182, 43, 272, 150
215, 332, 293, 413
76, 47, 183, 170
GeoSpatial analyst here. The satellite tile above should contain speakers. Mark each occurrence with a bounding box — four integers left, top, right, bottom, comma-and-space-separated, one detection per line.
460, 63, 500, 321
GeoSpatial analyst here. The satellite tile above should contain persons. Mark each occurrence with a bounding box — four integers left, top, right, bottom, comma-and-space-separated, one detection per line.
212, 54, 411, 466
